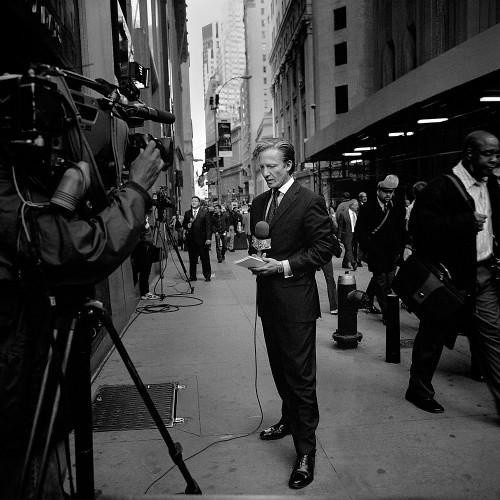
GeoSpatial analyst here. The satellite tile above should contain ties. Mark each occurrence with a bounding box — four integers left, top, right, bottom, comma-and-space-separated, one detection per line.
266, 190, 280, 226
194, 211, 197, 219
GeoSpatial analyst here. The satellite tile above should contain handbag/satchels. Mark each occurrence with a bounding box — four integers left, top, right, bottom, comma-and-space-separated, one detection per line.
390, 251, 470, 332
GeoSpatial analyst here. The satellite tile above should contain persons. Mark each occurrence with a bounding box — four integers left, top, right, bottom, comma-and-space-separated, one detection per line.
352, 174, 404, 322
401, 182, 429, 310
327, 207, 338, 228
337, 199, 359, 270
0, 139, 164, 500
356, 191, 368, 267
405, 130, 500, 425
248, 142, 334, 489
131, 196, 251, 301
456, 334, 486, 382
336, 192, 352, 223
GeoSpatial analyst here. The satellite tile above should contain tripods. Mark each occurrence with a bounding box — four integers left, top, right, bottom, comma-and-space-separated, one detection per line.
18, 296, 205, 498
151, 216, 195, 300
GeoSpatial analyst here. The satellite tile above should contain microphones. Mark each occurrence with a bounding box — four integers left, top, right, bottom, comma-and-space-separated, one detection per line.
189, 214, 195, 224
250, 221, 271, 256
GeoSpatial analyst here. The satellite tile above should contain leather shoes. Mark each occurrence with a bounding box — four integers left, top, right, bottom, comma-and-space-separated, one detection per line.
259, 422, 289, 441
187, 277, 198, 282
205, 276, 211, 282
288, 447, 317, 489
405, 386, 444, 414
366, 303, 381, 315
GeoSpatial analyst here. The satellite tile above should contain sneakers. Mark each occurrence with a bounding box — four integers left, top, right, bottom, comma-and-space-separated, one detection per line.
141, 292, 160, 301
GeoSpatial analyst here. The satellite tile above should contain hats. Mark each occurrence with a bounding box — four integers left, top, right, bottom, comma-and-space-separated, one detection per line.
377, 180, 397, 192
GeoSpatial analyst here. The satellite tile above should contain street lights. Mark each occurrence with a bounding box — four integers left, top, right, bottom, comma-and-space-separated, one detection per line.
208, 74, 254, 205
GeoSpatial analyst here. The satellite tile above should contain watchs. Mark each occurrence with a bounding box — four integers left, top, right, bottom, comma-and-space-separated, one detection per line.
276, 260, 283, 275
